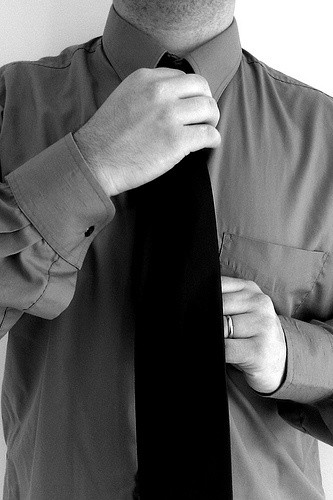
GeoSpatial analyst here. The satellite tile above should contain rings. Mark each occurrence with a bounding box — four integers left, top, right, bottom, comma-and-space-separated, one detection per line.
226, 315, 233, 339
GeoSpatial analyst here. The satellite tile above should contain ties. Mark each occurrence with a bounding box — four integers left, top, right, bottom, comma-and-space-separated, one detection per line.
125, 51, 232, 500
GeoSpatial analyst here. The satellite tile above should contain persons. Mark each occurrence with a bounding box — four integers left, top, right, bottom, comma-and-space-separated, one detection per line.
0, 0, 333, 500
